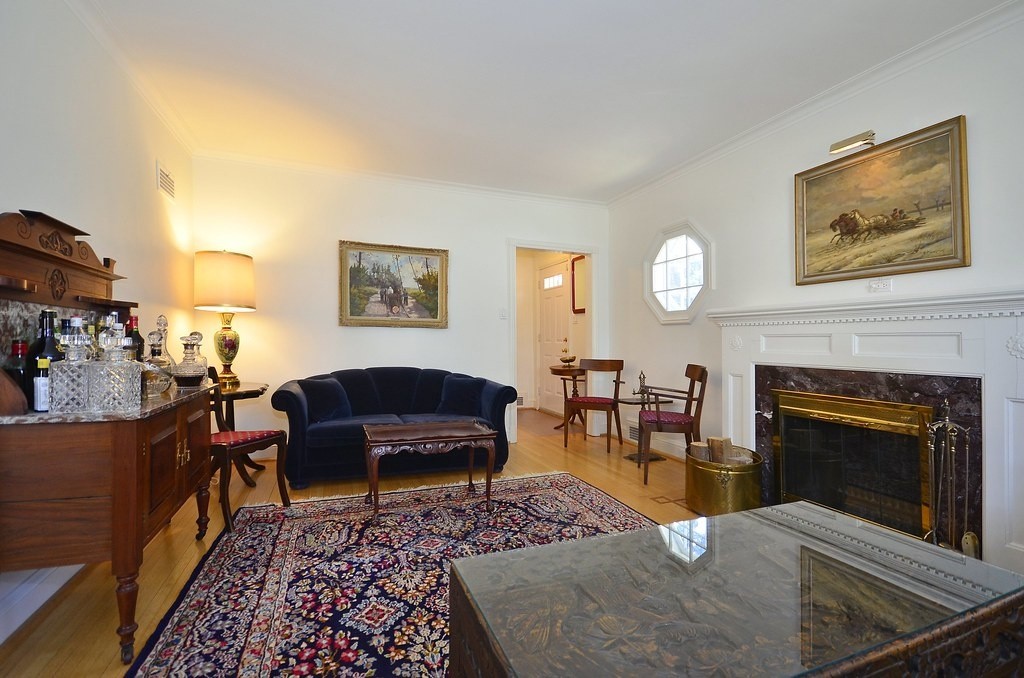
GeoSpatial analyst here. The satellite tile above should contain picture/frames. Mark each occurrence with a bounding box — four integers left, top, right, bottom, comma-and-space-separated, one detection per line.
794, 115, 971, 286
339, 240, 448, 329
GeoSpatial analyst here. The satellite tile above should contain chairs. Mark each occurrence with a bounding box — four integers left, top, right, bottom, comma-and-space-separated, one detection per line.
208, 367, 290, 532
638, 364, 708, 485
564, 359, 624, 453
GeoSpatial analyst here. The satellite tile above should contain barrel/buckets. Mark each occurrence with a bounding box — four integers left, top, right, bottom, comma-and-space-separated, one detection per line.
684, 443, 762, 515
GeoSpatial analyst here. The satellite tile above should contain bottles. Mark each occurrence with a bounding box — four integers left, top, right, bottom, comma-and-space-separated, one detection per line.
38, 308, 144, 363
49, 335, 91, 414
139, 354, 170, 402
27, 310, 61, 411
189, 332, 207, 386
33, 358, 51, 414
142, 315, 176, 366
144, 331, 173, 393
3, 339, 32, 409
88, 337, 141, 414
172, 336, 206, 389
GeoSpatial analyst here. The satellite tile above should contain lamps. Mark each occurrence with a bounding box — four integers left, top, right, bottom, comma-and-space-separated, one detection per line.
830, 130, 875, 154
193, 250, 256, 383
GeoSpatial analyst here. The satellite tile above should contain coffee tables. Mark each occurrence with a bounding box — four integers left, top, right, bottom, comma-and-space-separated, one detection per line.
363, 419, 498, 526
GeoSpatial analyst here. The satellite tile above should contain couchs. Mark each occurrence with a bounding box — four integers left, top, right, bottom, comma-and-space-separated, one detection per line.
271, 367, 517, 489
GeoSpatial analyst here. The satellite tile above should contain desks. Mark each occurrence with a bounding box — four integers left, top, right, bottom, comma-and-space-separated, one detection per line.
550, 365, 585, 429
210, 381, 269, 487
450, 501, 1024, 678
616, 398, 673, 462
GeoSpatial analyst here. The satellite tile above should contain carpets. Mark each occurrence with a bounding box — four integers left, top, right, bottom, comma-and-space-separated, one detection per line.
123, 470, 662, 678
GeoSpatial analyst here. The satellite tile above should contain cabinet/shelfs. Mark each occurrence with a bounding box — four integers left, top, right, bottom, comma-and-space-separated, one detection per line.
0, 209, 220, 665
